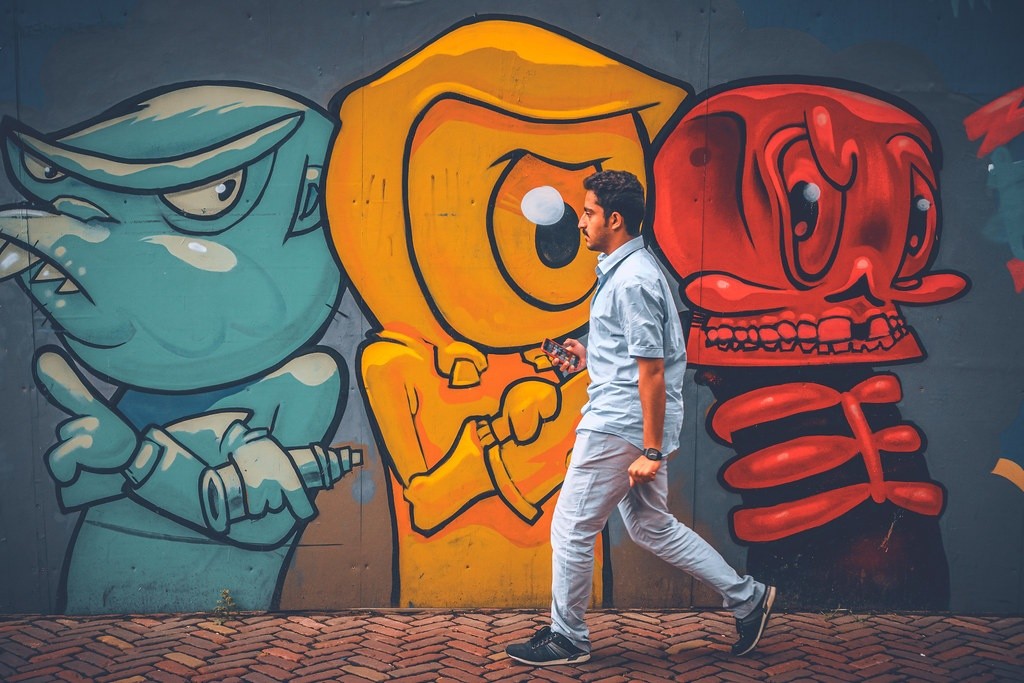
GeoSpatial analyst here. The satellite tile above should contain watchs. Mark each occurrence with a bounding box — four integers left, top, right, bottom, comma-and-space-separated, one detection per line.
642, 448, 663, 461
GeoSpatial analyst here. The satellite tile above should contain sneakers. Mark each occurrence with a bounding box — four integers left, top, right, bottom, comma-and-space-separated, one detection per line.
506, 626, 591, 666
733, 582, 777, 656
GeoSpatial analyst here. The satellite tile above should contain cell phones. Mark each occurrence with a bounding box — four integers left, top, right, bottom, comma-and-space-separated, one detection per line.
541, 337, 581, 370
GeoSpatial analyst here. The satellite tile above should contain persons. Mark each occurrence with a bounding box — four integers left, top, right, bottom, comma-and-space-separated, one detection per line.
504, 169, 776, 666
